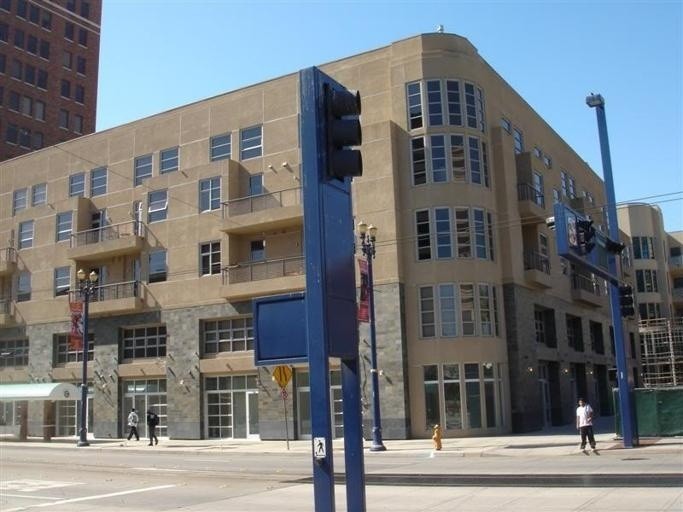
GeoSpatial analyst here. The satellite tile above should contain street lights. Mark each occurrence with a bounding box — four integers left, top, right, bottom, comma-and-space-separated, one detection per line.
356, 219, 386, 452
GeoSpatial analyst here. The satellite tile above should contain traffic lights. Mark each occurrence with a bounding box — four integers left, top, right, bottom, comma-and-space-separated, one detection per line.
77, 267, 99, 447
577, 219, 596, 254
620, 282, 635, 318
323, 81, 364, 182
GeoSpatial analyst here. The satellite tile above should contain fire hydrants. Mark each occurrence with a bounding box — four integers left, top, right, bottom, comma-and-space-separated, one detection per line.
432, 423, 441, 450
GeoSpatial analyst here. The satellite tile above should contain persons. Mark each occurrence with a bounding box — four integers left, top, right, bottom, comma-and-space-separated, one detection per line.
147, 409, 158, 445
576, 397, 595, 452
127, 409, 139, 440
